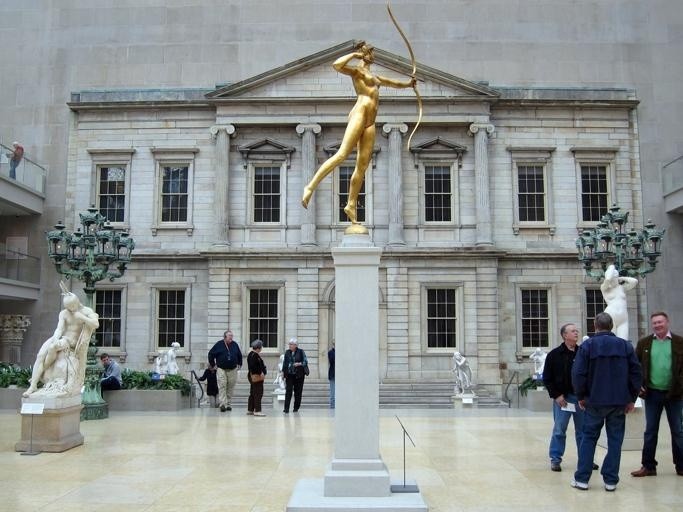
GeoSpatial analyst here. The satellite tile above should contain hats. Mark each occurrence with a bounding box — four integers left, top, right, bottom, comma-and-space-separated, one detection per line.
252, 340, 264, 348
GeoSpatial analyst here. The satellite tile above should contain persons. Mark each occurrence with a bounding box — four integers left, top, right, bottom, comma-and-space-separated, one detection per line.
154, 351, 162, 373
208, 331, 241, 412
281, 338, 308, 413
302, 43, 417, 224
570, 311, 642, 491
22, 292, 99, 398
166, 342, 180, 375
542, 323, 598, 471
7, 142, 23, 180
453, 352, 471, 394
247, 339, 266, 416
101, 352, 123, 396
529, 347, 547, 373
599, 264, 638, 340
328, 339, 334, 408
198, 363, 219, 408
630, 312, 682, 477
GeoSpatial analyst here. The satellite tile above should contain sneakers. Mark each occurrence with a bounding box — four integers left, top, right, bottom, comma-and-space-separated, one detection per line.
569, 479, 589, 490
293, 408, 298, 412
221, 406, 232, 411
604, 483, 617, 491
247, 411, 266, 416
283, 409, 290, 413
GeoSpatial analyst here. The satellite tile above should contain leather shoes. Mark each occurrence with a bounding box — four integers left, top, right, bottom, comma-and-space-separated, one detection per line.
677, 470, 683, 476
631, 467, 657, 476
551, 462, 561, 471
593, 463, 599, 470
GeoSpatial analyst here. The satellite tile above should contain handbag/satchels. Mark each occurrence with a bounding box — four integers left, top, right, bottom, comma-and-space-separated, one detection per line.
251, 371, 264, 382
304, 366, 309, 376
